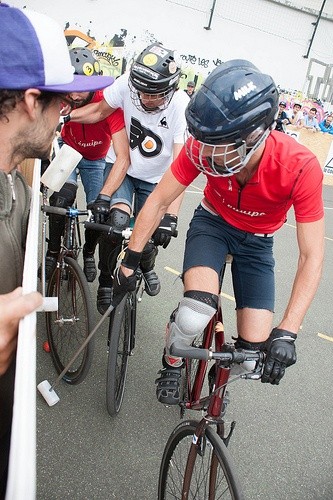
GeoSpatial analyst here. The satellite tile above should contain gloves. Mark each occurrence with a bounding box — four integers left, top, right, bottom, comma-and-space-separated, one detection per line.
111, 268, 137, 306
261, 329, 297, 385
41, 159, 52, 192
88, 193, 111, 223
150, 213, 178, 249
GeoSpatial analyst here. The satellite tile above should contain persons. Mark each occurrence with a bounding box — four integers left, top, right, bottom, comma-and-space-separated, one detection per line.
111, 59, 327, 406
0, 1, 113, 382
38, 47, 131, 284
183, 80, 197, 97
57, 40, 193, 316
303, 107, 322, 133
274, 101, 291, 133
318, 114, 333, 135
285, 102, 305, 132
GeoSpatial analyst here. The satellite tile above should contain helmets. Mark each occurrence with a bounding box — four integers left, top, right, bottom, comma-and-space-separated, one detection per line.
127, 40, 183, 115
69, 46, 100, 79
184, 56, 282, 178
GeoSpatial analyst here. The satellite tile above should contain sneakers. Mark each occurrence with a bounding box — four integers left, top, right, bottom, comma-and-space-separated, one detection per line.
155, 362, 181, 405
138, 265, 161, 296
37, 256, 56, 281
93, 286, 114, 316
79, 256, 97, 283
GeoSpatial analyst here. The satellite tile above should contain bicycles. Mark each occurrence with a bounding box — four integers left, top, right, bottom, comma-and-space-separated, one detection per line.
82, 188, 179, 415
36, 167, 101, 389
158, 252, 281, 500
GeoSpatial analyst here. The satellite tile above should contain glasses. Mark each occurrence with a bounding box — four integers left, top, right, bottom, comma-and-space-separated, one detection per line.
57, 92, 75, 116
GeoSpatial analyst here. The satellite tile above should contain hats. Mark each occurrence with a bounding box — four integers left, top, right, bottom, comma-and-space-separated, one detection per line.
186, 80, 195, 87
0, 3, 115, 92
279, 101, 286, 107
